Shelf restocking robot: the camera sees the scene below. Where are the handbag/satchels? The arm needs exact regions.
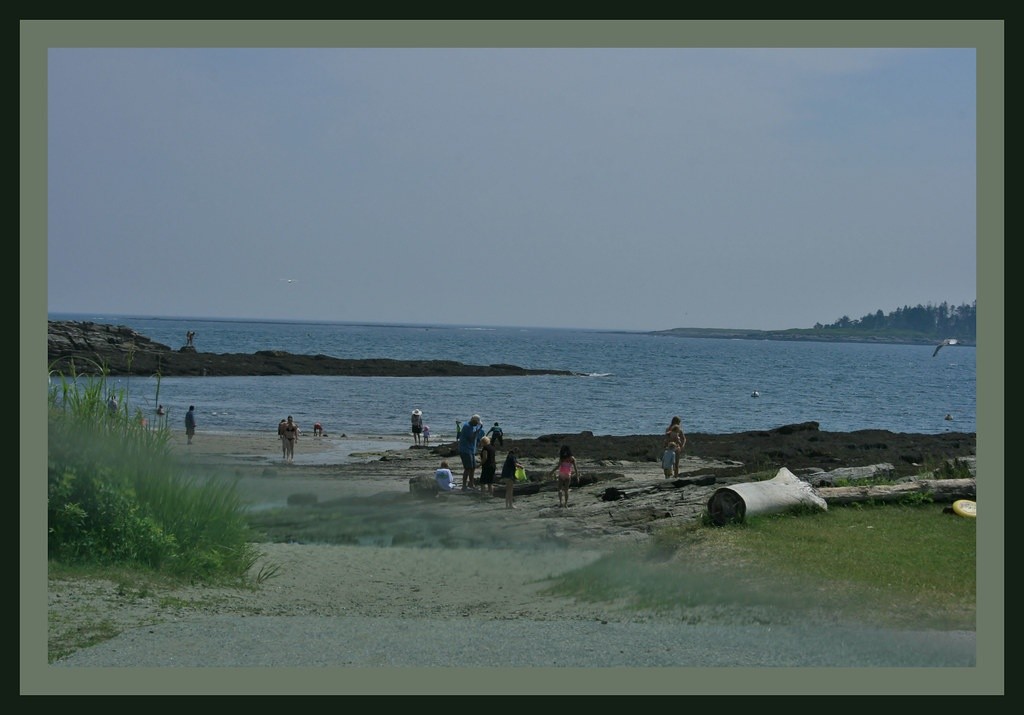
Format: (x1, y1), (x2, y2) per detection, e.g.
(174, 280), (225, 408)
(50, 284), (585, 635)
(515, 467), (527, 482)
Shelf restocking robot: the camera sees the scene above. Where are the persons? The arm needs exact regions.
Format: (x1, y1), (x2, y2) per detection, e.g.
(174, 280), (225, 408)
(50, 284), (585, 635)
(108, 396), (118, 426)
(411, 409), (504, 498)
(157, 405), (165, 415)
(313, 422), (323, 437)
(501, 447), (524, 509)
(186, 331), (195, 346)
(662, 416), (686, 479)
(549, 445), (580, 508)
(185, 405), (197, 445)
(278, 415), (302, 462)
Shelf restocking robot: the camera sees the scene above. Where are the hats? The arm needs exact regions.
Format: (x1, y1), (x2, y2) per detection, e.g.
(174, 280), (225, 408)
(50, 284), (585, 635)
(481, 436), (490, 446)
(423, 426), (430, 431)
(471, 414), (482, 425)
(666, 442), (680, 450)
(514, 447), (522, 459)
(412, 409), (422, 415)
(672, 424), (680, 433)
